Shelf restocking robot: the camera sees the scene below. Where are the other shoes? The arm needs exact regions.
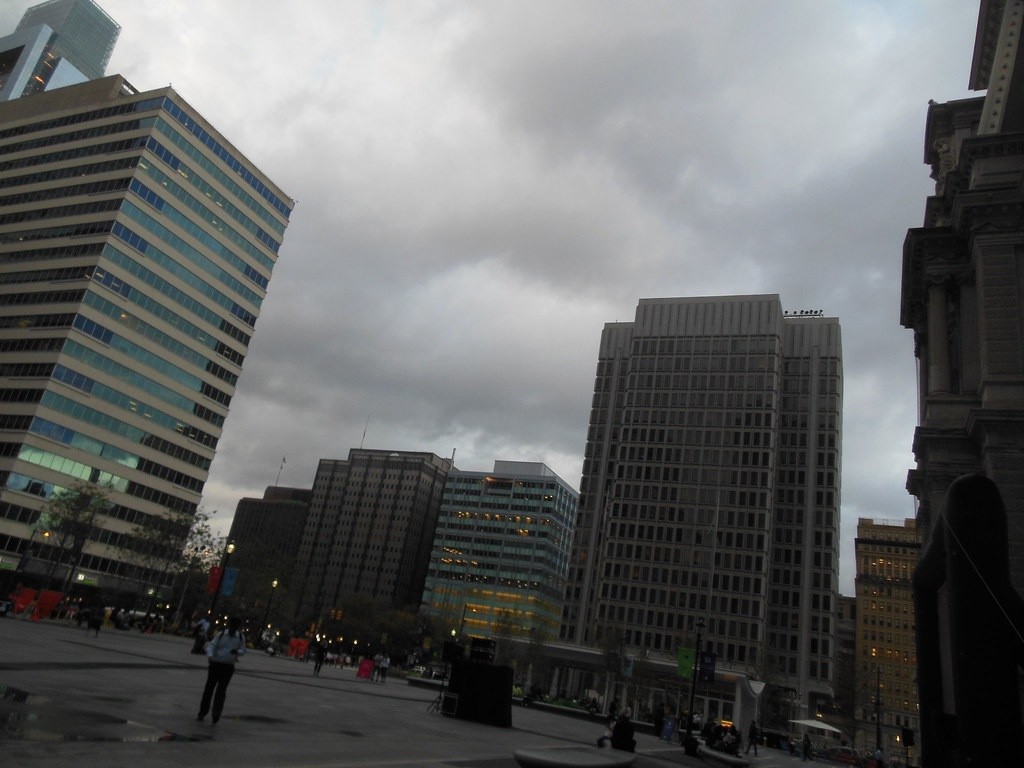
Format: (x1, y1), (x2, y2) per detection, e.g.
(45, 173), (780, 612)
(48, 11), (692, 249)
(197, 714), (204, 721)
(213, 718), (218, 724)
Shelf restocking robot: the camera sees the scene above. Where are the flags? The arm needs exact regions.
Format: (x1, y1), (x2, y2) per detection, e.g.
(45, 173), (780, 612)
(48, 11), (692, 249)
(288, 638), (312, 658)
(31, 588), (64, 621)
(208, 566), (223, 592)
(15, 588), (36, 610)
(222, 566), (240, 594)
(356, 658), (376, 680)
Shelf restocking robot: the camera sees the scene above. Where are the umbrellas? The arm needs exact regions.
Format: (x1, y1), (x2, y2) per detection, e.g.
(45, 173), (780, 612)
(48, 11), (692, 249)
(786, 717), (843, 750)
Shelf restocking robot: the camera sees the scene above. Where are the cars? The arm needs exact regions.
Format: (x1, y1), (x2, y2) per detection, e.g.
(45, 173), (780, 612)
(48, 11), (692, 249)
(758, 729), (796, 751)
(410, 665), (424, 674)
(821, 746), (860, 763)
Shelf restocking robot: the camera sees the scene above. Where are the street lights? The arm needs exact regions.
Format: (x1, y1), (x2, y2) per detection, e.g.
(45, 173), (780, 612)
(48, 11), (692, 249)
(209, 542), (235, 621)
(2, 530), (49, 601)
(687, 620), (706, 737)
(876, 670), (884, 750)
(258, 580), (278, 645)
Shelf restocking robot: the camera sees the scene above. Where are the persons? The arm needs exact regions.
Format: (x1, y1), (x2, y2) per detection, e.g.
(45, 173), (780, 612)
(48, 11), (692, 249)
(197, 615), (246, 724)
(0, 582), (390, 683)
(403, 663), (886, 768)
